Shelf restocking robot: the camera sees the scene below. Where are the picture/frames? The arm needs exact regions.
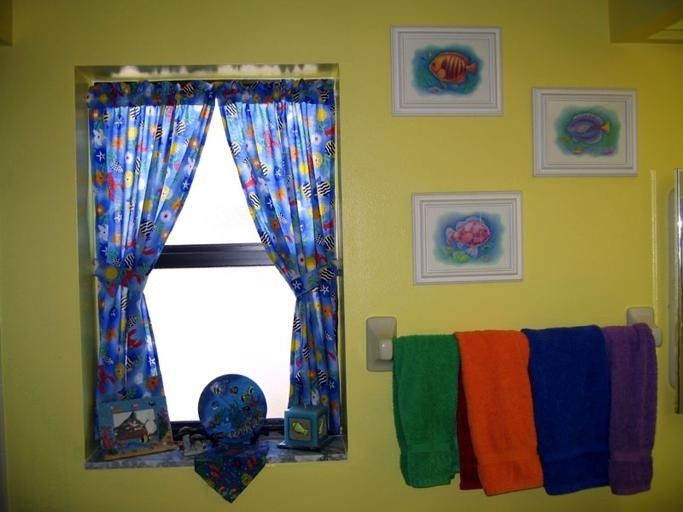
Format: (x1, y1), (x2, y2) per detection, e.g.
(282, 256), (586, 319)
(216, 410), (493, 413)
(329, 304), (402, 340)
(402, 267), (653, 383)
(532, 88), (637, 177)
(413, 191), (523, 284)
(96, 396), (176, 461)
(391, 26), (503, 116)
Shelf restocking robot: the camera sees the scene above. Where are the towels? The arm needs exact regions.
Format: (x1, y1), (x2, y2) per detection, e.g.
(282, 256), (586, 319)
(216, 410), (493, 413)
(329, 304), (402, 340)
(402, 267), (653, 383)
(600, 323), (658, 494)
(452, 330), (544, 496)
(520, 324), (611, 496)
(395, 332), (461, 488)
